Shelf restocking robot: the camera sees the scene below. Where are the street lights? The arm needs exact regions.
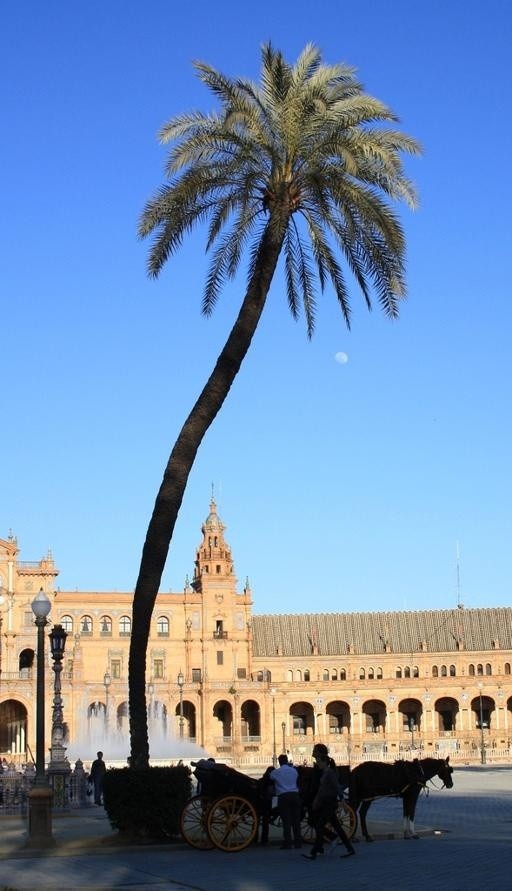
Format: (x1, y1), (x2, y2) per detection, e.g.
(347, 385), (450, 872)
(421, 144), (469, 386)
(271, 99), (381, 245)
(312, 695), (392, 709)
(178, 667), (185, 738)
(476, 679), (488, 764)
(281, 721), (287, 753)
(102, 669), (111, 733)
(23, 586), (61, 848)
(46, 623), (74, 811)
(147, 678), (155, 722)
(268, 687), (279, 769)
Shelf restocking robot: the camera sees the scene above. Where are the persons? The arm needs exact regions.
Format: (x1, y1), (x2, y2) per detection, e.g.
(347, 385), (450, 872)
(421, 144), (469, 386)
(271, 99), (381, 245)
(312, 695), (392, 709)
(268, 754), (303, 850)
(177, 760), (184, 767)
(300, 754), (357, 862)
(2, 758), (10, 773)
(1, 757), (5, 775)
(251, 765), (276, 843)
(87, 751), (109, 808)
(196, 757), (216, 827)
(306, 743), (340, 859)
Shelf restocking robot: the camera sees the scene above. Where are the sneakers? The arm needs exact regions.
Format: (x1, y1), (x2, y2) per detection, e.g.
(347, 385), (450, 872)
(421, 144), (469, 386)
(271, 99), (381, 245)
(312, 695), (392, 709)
(94, 799), (104, 807)
(340, 851), (355, 858)
(302, 854), (316, 860)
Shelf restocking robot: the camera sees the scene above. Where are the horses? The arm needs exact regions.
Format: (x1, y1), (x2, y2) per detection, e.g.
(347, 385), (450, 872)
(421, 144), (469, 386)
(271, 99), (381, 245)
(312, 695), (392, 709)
(349, 756), (453, 842)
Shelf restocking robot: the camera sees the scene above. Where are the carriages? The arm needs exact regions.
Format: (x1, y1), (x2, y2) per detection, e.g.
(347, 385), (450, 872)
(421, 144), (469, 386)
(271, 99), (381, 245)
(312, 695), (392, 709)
(178, 754), (454, 854)
